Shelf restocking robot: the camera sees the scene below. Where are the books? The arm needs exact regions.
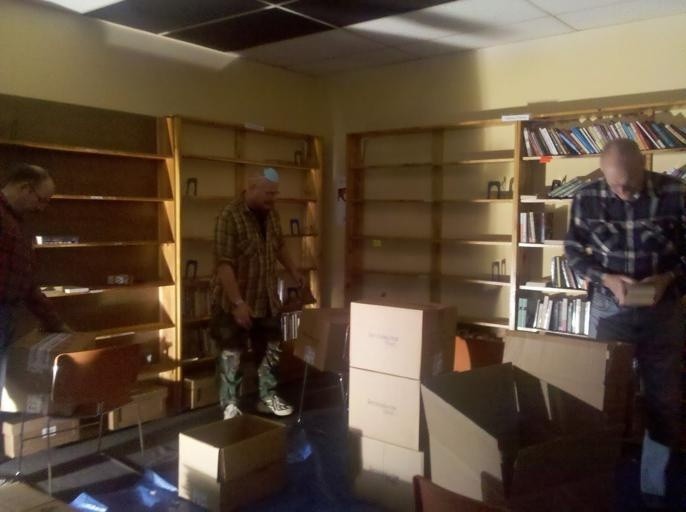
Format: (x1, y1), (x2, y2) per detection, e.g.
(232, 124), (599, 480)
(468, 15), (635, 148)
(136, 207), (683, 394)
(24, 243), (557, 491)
(522, 118), (686, 156)
(663, 167), (686, 182)
(515, 176), (601, 336)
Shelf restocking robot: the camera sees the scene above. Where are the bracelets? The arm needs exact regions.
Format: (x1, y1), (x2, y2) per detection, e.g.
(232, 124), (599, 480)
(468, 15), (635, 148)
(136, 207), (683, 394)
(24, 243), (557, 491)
(664, 270), (677, 280)
(230, 299), (244, 309)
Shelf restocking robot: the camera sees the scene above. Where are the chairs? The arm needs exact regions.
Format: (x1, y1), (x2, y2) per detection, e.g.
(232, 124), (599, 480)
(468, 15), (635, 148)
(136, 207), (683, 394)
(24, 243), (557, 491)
(12, 345), (146, 500)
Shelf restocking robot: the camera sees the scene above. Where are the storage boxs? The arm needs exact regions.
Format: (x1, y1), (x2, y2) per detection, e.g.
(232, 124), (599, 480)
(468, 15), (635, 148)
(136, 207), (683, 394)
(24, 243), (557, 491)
(1, 478), (85, 512)
(176, 412), (288, 511)
(345, 293), (633, 511)
(0, 326), (99, 418)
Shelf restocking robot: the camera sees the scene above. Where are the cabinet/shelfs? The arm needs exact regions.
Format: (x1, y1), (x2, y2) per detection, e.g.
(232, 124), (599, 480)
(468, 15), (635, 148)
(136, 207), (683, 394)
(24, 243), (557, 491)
(517, 97), (685, 341)
(175, 113), (323, 411)
(0, 92), (180, 458)
(343, 117), (518, 332)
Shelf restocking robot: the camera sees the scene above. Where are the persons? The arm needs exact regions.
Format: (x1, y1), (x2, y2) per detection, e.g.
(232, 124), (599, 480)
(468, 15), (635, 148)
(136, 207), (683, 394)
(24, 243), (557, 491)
(0, 164), (73, 398)
(561, 135), (686, 474)
(207, 171), (306, 421)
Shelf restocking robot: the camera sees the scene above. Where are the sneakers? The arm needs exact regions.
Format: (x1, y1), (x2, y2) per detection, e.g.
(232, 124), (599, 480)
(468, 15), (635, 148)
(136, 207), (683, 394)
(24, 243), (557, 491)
(255, 393), (295, 416)
(223, 403), (243, 421)
